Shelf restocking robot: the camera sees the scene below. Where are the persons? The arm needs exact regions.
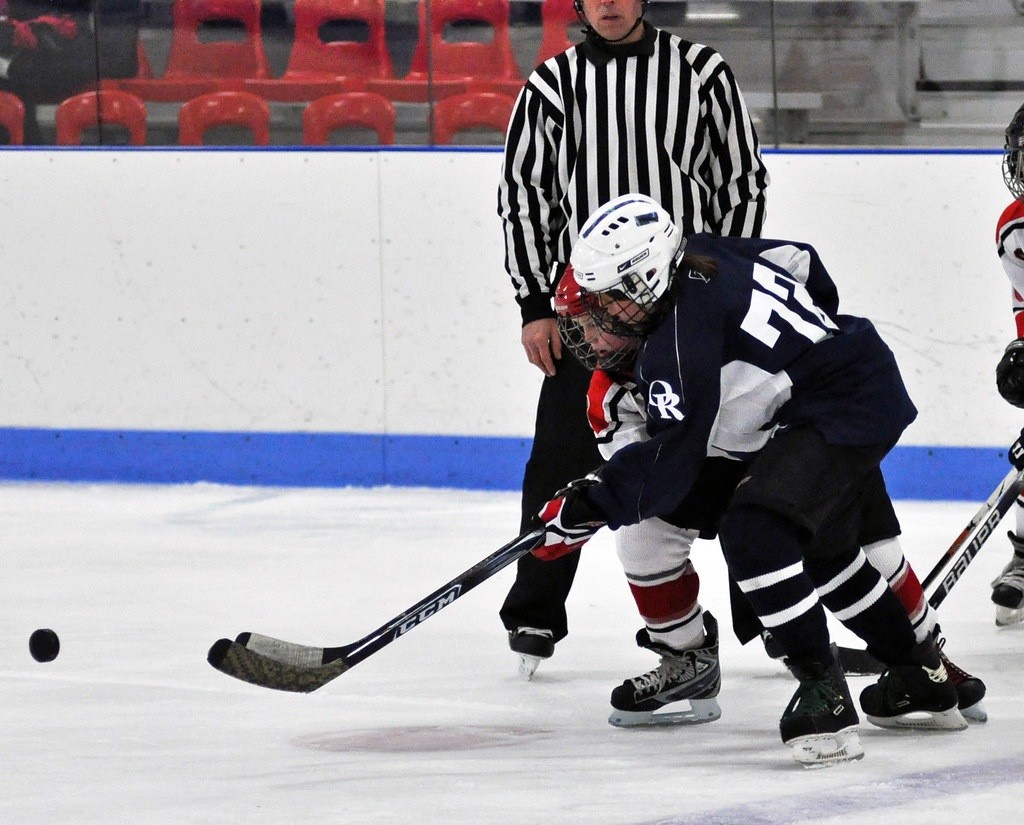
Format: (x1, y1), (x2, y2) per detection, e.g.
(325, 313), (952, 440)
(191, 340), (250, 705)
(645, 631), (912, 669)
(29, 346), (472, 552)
(498, 2), (771, 680)
(0, 0), (139, 146)
(991, 104), (1024, 627)
(533, 194), (990, 768)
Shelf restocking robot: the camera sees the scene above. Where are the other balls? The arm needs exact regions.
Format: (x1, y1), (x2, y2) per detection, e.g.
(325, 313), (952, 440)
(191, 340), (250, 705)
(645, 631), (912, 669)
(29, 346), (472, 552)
(28, 629), (59, 663)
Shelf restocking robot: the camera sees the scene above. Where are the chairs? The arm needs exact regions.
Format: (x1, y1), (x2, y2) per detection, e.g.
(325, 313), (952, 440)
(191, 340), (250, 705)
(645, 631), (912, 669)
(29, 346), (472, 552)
(0, 0), (589, 146)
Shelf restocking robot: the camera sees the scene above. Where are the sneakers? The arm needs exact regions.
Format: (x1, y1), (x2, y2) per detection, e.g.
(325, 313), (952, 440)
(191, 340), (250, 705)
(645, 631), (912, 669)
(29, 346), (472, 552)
(608, 610), (722, 728)
(858, 621), (969, 730)
(990, 531), (1024, 626)
(508, 625), (555, 682)
(779, 646), (864, 769)
(924, 623), (989, 722)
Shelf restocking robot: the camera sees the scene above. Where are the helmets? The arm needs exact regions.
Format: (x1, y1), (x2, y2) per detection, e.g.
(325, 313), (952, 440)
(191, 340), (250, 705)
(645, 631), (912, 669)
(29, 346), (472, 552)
(569, 194), (687, 336)
(556, 267), (641, 371)
(1001, 102), (1024, 202)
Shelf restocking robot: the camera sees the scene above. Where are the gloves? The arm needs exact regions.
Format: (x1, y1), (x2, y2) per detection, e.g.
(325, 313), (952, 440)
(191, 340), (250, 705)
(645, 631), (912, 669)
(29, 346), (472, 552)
(995, 336), (1024, 412)
(530, 467), (607, 562)
(1008, 426), (1024, 473)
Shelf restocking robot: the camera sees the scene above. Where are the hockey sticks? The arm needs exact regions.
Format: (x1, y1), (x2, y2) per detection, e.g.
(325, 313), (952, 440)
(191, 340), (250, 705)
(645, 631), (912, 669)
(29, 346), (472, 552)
(208, 523), (549, 694)
(782, 465), (1021, 679)
(236, 518), (546, 667)
(832, 472), (1024, 674)
